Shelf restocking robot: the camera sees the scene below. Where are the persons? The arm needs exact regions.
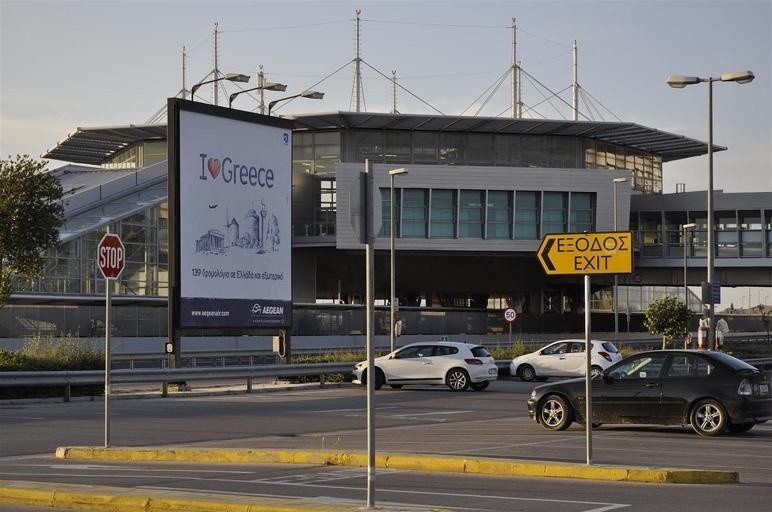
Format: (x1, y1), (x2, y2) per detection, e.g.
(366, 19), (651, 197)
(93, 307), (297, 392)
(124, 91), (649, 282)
(682, 314), (729, 350)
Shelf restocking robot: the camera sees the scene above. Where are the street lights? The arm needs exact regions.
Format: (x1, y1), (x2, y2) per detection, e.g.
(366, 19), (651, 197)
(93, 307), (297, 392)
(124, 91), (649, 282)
(613, 175), (629, 347)
(661, 68), (756, 357)
(385, 167), (413, 357)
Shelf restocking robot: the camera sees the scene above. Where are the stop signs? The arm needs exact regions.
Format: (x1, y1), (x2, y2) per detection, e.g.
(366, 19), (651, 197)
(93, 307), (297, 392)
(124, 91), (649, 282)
(95, 232), (127, 282)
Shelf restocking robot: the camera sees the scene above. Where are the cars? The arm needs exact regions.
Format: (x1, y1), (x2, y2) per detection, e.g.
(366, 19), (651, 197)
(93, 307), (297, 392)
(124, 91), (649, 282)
(349, 339), (501, 394)
(525, 347), (772, 439)
(508, 337), (625, 385)
